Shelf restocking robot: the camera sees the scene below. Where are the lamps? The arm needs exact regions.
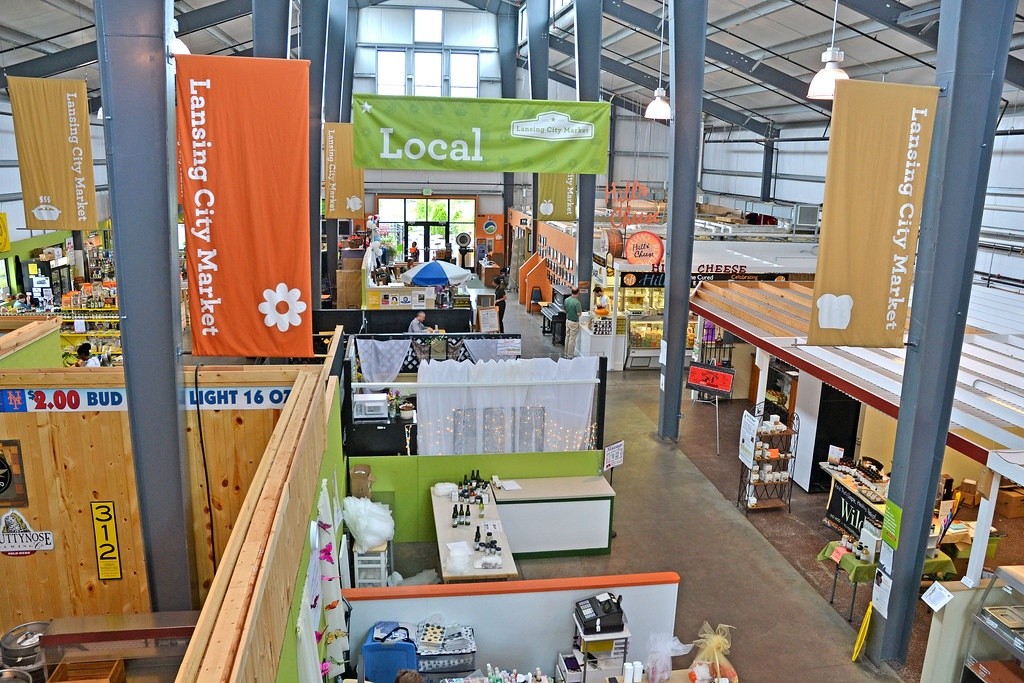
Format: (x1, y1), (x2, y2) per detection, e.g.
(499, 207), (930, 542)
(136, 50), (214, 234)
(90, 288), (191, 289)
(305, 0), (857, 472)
(172, 20), (193, 74)
(643, 0), (671, 119)
(97, 107), (103, 119)
(804, 0), (850, 99)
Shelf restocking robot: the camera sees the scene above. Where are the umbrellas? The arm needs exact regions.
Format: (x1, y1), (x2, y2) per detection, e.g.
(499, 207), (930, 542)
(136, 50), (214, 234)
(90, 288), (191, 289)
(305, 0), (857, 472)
(401, 259), (471, 286)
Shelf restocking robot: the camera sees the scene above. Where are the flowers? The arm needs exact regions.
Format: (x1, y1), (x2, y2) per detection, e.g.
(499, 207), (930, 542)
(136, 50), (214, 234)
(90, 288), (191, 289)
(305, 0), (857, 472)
(389, 389), (414, 410)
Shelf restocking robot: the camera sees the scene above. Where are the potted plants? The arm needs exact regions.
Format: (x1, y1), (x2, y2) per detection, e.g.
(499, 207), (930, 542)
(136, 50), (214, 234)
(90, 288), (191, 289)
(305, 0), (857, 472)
(386, 248), (399, 266)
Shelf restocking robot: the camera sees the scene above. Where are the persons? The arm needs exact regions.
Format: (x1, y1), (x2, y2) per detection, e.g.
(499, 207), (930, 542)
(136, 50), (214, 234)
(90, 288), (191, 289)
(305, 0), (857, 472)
(395, 670), (423, 683)
(410, 242), (420, 262)
(77, 343), (101, 367)
(593, 287), (610, 310)
(492, 277), (507, 333)
(14, 293), (31, 310)
(408, 311), (433, 333)
(564, 288), (582, 359)
(436, 285), (443, 293)
(403, 259), (414, 272)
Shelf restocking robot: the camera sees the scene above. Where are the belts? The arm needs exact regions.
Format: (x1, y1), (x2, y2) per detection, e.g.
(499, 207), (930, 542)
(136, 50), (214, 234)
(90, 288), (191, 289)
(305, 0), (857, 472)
(567, 318), (578, 322)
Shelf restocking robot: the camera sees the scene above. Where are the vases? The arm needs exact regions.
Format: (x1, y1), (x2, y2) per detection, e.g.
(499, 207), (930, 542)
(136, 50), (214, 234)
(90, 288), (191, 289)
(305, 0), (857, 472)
(389, 399), (413, 420)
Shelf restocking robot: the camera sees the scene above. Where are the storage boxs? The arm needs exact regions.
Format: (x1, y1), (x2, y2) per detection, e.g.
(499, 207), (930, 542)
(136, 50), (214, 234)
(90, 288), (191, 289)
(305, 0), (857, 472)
(938, 474), (1023, 559)
(46, 656), (127, 683)
(350, 464), (375, 497)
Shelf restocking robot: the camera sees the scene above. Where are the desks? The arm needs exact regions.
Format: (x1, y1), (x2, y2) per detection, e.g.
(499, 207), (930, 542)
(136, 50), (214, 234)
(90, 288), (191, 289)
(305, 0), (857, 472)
(39, 610), (202, 683)
(429, 475), (614, 584)
(479, 260), (500, 287)
(818, 462), (960, 622)
(343, 396), (418, 456)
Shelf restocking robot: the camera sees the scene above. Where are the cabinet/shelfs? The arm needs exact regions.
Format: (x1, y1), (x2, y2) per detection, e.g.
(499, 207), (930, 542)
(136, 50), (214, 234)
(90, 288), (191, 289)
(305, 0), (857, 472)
(351, 538), (389, 589)
(62, 248), (121, 366)
(579, 284), (697, 369)
(554, 612), (632, 683)
(959, 564), (1024, 682)
(737, 401), (800, 516)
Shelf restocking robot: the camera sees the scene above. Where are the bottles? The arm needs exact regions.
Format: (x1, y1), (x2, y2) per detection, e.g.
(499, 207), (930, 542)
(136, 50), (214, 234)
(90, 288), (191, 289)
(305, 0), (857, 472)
(837, 456), (883, 492)
(458, 469), (490, 504)
(119, 336), (122, 348)
(452, 504), (470, 527)
(842, 516), (883, 563)
(475, 526), (501, 555)
(87, 296), (102, 309)
(479, 498), (484, 518)
